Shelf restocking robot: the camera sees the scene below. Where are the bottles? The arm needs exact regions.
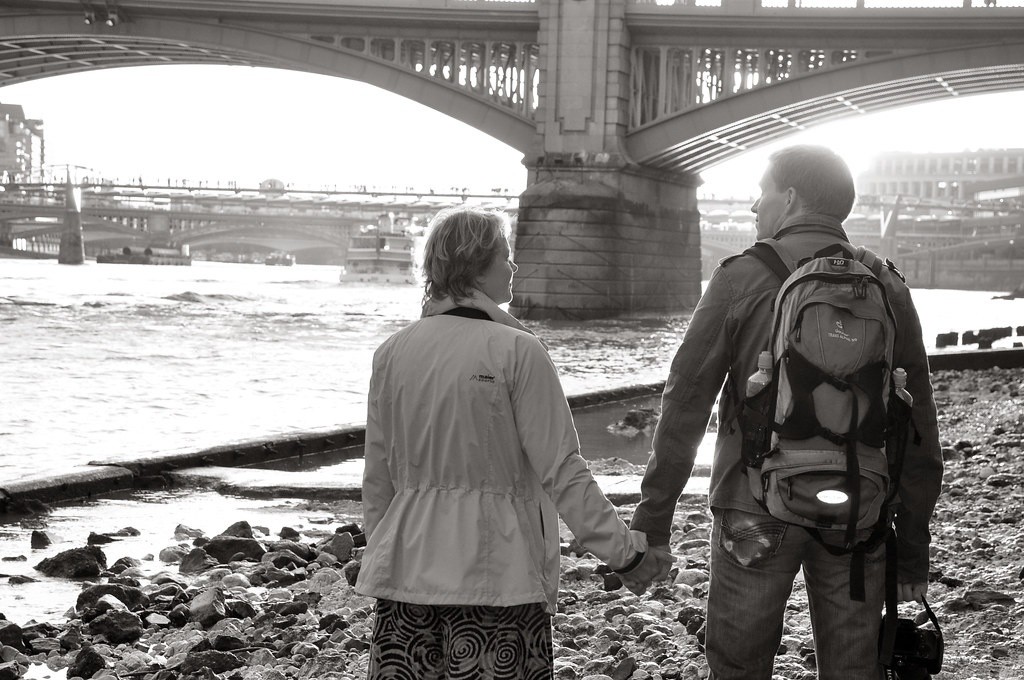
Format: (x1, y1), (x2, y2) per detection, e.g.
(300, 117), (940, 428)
(742, 351), (774, 468)
(887, 367), (913, 466)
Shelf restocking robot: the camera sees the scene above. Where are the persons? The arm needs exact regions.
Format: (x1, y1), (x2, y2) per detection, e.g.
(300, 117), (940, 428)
(352, 209), (679, 680)
(620, 144), (944, 680)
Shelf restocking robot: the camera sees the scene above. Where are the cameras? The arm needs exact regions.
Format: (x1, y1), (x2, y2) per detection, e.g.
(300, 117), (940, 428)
(878, 593), (944, 680)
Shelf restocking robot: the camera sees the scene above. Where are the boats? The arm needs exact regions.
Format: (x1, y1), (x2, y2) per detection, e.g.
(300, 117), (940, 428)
(264, 254), (296, 267)
(338, 214), (428, 288)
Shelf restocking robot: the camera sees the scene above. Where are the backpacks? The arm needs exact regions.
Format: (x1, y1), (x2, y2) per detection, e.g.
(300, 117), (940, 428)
(743, 241), (905, 528)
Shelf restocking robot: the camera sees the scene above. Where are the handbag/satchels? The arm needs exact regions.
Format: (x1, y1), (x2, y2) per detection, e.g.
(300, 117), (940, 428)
(891, 595), (945, 675)
